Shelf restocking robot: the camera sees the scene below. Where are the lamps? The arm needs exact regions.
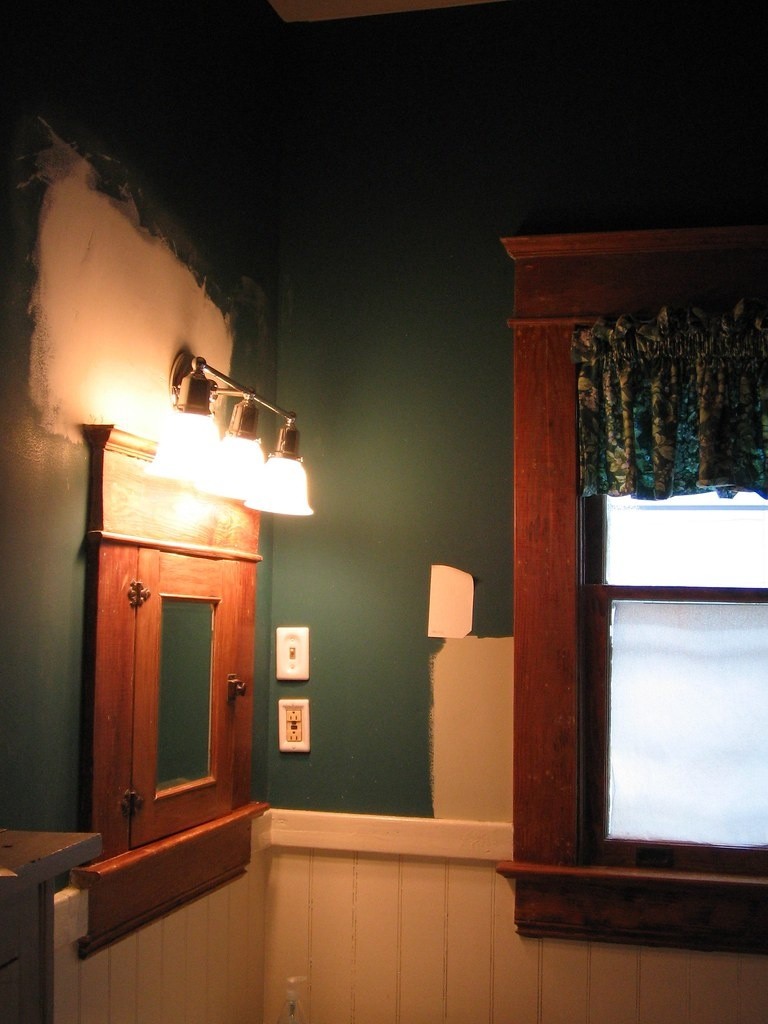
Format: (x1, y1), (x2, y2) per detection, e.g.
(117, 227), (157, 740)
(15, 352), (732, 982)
(144, 358), (319, 519)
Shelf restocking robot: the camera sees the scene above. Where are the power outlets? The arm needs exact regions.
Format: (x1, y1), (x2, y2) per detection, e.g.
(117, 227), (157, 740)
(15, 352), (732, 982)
(276, 698), (312, 754)
